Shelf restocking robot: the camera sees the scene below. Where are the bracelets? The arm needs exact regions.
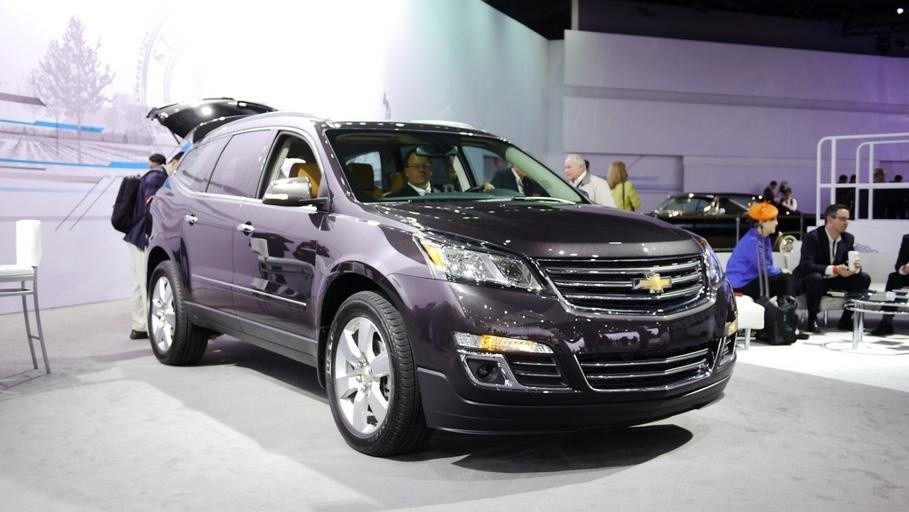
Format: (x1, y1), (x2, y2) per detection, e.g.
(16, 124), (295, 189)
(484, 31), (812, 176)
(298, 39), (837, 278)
(833, 265), (838, 274)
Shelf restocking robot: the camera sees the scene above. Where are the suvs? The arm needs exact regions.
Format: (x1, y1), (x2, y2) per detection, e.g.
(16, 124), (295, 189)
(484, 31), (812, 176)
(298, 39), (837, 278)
(141, 97), (738, 457)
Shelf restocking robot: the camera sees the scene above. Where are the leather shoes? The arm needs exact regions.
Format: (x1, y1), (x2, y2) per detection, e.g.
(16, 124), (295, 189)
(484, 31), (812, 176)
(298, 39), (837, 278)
(130, 330), (149, 340)
(796, 331), (809, 339)
(807, 320), (820, 334)
(872, 322), (894, 335)
(838, 319), (853, 331)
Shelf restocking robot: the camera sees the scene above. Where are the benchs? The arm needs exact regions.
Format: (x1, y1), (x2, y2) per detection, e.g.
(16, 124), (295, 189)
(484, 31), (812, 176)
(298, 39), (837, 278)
(716, 250), (909, 349)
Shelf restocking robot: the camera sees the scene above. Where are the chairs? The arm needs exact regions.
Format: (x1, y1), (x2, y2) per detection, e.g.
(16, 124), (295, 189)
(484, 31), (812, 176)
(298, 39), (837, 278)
(347, 163), (383, 199)
(382, 172), (407, 199)
(289, 164), (321, 198)
(0, 220), (52, 374)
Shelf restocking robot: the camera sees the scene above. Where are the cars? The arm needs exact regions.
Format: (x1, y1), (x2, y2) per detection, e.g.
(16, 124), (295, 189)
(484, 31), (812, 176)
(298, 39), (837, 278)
(642, 192), (825, 253)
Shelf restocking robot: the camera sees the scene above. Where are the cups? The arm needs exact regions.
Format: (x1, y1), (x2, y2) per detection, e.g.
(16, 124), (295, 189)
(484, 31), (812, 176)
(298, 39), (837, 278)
(847, 250), (861, 274)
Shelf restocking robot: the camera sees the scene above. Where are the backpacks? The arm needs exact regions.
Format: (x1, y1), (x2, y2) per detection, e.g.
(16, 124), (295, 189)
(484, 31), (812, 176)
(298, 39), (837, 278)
(111, 170), (163, 233)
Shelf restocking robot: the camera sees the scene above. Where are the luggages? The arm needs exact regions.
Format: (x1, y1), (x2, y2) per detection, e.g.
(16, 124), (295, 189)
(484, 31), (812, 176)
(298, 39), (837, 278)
(755, 222), (798, 345)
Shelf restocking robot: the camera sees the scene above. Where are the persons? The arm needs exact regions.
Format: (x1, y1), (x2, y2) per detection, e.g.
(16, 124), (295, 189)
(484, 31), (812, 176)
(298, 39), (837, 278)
(491, 163), (535, 197)
(563, 154), (616, 208)
(836, 168), (909, 219)
(385, 148), (495, 197)
(763, 180), (797, 212)
(870, 233), (909, 336)
(149, 152), (166, 169)
(123, 151), (186, 341)
(792, 204), (871, 333)
(607, 160), (639, 212)
(723, 202), (809, 341)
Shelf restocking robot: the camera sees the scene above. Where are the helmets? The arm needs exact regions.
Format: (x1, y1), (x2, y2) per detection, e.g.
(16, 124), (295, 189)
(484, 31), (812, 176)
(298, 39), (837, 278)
(748, 202), (779, 222)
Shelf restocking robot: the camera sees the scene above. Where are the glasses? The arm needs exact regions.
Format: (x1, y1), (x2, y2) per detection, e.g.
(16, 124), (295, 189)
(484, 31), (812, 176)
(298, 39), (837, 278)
(408, 162), (431, 170)
